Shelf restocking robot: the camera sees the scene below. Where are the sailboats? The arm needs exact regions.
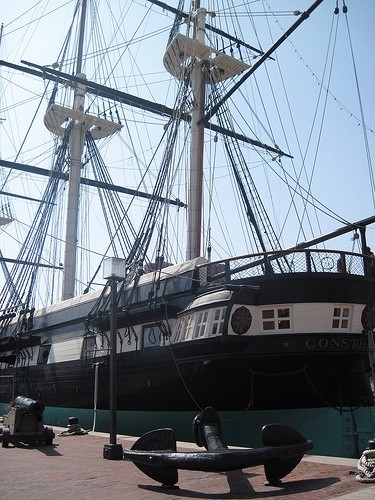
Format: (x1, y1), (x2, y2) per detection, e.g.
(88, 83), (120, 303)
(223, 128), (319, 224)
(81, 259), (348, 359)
(0, 0), (375, 403)
